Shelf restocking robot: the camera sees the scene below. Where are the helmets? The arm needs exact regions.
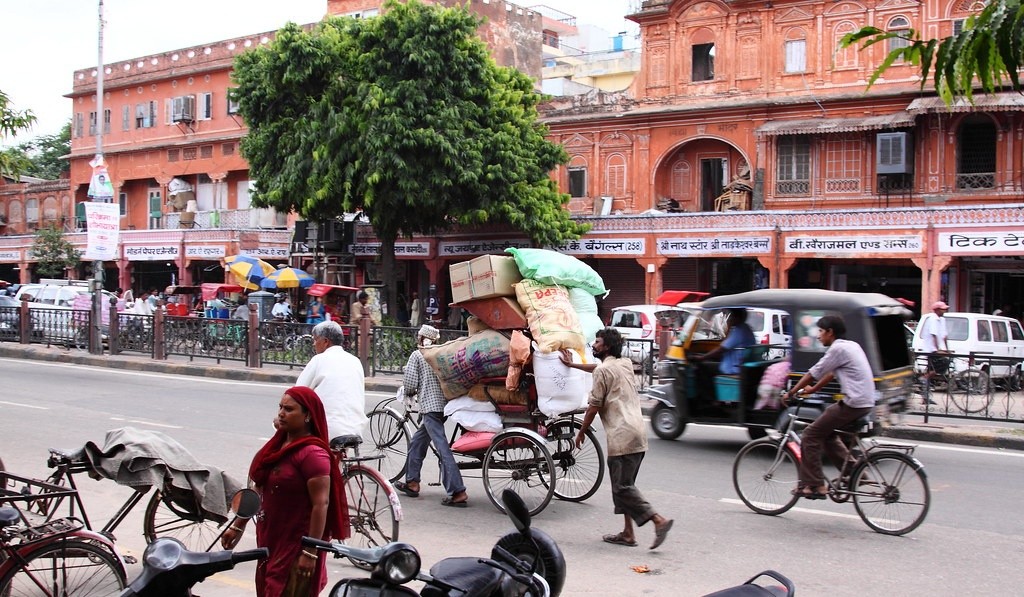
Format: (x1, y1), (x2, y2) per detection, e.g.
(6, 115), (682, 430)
(275, 292), (287, 302)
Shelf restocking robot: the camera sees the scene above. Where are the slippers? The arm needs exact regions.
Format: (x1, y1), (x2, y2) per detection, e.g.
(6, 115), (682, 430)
(603, 533), (638, 546)
(441, 496), (467, 506)
(395, 480), (420, 497)
(650, 520), (673, 550)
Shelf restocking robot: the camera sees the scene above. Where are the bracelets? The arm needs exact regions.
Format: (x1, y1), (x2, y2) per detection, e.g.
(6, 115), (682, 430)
(229, 525), (244, 533)
(788, 390), (793, 396)
(301, 549), (319, 559)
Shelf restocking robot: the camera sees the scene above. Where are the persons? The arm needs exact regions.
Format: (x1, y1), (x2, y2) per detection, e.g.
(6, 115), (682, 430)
(912, 301), (951, 405)
(989, 305), (1010, 342)
(559, 329), (674, 550)
(109, 286), (460, 352)
(781, 315), (875, 501)
(688, 306), (758, 417)
(221, 386), (351, 597)
(273, 321), (370, 545)
(393, 324), (468, 507)
(96, 175), (111, 195)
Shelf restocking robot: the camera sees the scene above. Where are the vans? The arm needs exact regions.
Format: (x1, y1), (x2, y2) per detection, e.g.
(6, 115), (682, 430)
(12, 279), (145, 351)
(910, 312), (1024, 391)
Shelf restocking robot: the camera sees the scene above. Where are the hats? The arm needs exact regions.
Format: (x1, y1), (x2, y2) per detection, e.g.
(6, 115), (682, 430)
(933, 301), (949, 309)
(992, 309), (1003, 315)
(418, 324), (440, 340)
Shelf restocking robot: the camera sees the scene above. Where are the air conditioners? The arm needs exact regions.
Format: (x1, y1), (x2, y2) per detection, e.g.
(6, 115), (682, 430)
(172, 96), (193, 122)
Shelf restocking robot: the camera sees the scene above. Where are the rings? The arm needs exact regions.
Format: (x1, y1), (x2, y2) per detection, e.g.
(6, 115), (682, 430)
(306, 571), (311, 577)
(297, 570), (302, 575)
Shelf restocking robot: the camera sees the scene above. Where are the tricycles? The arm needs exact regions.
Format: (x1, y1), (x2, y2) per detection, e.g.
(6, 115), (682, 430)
(354, 378), (605, 519)
(647, 287), (913, 445)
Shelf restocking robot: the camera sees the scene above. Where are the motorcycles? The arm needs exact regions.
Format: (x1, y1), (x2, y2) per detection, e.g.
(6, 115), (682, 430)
(477, 489), (795, 597)
(300, 528), (567, 597)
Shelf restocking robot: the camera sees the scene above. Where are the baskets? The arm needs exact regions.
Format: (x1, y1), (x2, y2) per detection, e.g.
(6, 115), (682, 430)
(932, 357), (950, 372)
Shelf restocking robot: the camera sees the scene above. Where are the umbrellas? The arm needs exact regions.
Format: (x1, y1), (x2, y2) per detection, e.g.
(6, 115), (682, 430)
(220, 254), (316, 313)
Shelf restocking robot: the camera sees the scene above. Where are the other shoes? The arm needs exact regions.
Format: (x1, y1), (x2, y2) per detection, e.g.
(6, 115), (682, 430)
(923, 399), (937, 404)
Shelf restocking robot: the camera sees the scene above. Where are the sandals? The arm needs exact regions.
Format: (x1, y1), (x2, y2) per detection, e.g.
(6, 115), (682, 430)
(792, 485), (826, 499)
(842, 474), (869, 486)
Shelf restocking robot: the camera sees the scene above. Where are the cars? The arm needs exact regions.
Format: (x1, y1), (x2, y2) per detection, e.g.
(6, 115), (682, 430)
(903, 323), (915, 351)
(742, 307), (793, 360)
(0, 295), (46, 343)
(607, 304), (695, 376)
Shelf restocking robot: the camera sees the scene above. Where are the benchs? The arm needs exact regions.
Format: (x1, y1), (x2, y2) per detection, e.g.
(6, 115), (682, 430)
(712, 345), (788, 403)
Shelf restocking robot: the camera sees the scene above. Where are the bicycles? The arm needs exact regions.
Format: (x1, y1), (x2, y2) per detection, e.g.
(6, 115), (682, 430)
(72, 310), (317, 357)
(0, 514), (129, 597)
(908, 347), (995, 414)
(22, 443), (243, 554)
(327, 433), (404, 571)
(732, 378), (931, 535)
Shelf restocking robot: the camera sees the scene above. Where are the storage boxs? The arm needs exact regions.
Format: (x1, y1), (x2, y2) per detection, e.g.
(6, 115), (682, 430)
(449, 254), (521, 303)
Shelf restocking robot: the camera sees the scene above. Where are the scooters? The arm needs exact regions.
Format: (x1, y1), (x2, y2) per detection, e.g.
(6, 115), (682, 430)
(115, 489), (270, 597)
